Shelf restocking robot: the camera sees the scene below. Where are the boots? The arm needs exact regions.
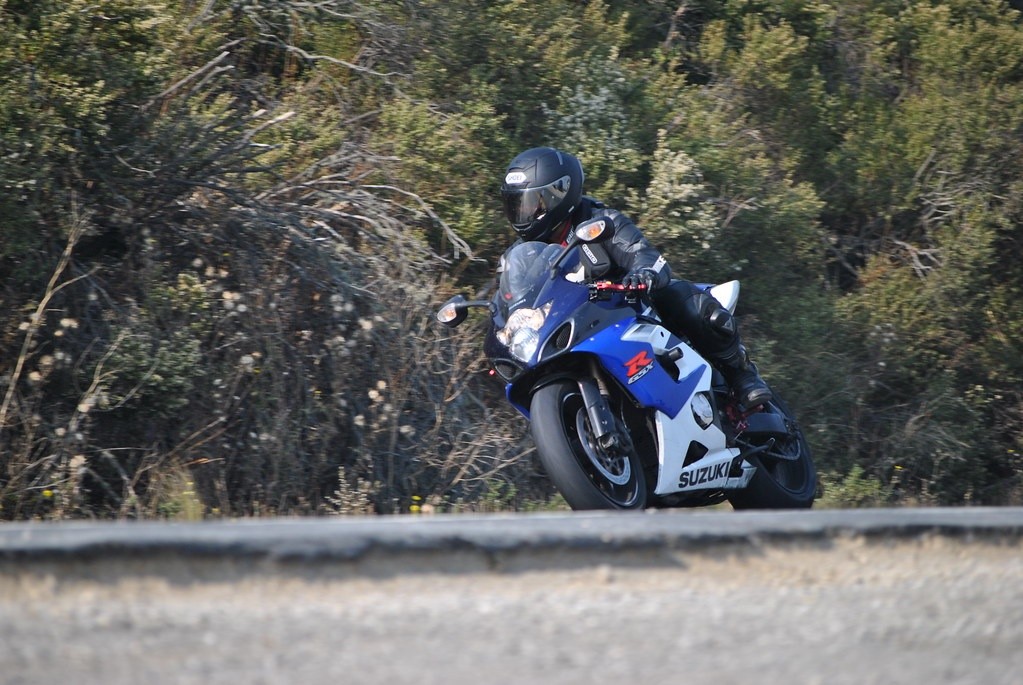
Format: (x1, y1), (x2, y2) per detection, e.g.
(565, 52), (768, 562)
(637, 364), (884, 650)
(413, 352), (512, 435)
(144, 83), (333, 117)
(704, 330), (773, 409)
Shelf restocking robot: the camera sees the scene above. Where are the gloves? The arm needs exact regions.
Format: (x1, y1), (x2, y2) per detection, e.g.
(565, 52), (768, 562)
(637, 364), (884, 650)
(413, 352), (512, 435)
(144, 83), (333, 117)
(622, 267), (660, 296)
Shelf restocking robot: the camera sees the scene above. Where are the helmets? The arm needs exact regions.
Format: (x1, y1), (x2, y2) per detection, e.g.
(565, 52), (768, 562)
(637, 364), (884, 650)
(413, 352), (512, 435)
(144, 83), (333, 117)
(500, 146), (586, 242)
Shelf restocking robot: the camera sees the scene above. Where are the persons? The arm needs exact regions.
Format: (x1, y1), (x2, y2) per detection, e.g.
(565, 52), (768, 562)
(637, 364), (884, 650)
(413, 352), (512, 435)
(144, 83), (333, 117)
(479, 147), (772, 405)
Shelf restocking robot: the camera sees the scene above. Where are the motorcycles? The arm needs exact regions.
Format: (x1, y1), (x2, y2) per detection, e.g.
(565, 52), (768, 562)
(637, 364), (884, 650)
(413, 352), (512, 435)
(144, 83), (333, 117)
(436, 216), (816, 509)
